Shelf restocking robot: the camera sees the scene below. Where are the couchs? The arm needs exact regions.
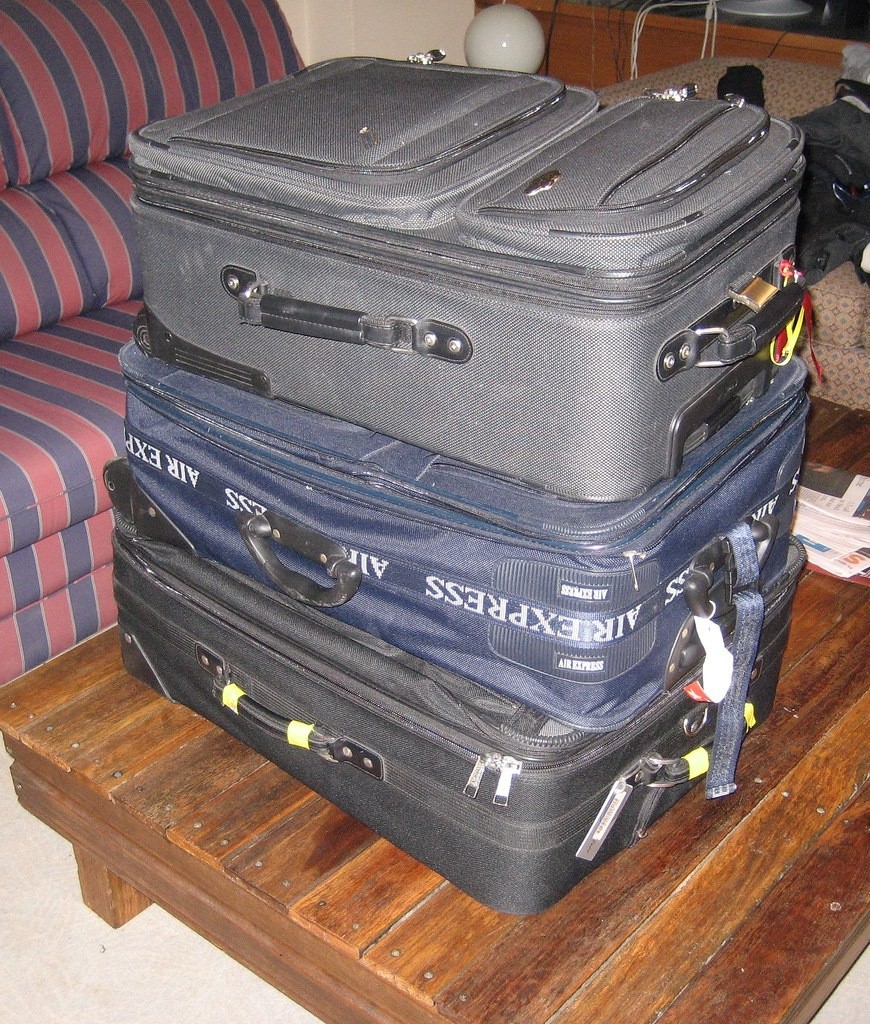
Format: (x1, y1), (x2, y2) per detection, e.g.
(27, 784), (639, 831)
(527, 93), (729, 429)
(0, 0), (339, 688)
(594, 54), (870, 417)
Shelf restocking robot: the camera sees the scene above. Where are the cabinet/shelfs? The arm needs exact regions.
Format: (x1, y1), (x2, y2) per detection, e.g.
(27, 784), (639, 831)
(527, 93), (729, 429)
(472, 0), (870, 96)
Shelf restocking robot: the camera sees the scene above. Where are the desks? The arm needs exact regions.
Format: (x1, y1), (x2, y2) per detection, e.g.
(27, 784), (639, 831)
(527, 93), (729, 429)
(0, 392), (870, 1024)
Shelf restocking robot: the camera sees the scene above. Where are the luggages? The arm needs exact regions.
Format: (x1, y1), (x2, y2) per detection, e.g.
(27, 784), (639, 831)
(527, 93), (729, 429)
(110, 531), (796, 918)
(127, 57), (807, 492)
(120, 347), (806, 731)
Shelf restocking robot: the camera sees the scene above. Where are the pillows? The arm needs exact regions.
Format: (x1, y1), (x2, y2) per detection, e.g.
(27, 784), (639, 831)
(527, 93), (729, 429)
(0, 0), (306, 347)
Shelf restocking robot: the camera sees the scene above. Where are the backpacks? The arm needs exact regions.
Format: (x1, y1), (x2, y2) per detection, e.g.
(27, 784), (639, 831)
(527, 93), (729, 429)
(790, 78), (870, 280)
